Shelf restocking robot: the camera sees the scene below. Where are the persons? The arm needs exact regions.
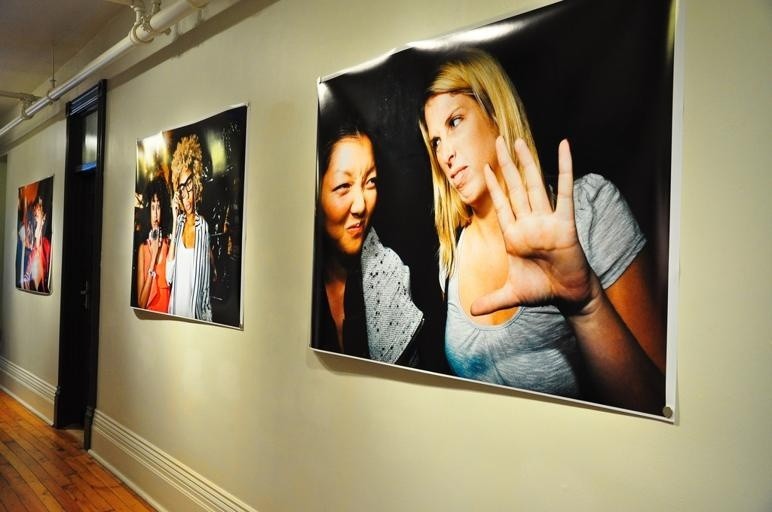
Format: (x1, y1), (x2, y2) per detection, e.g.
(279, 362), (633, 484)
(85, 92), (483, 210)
(135, 174), (174, 315)
(415, 45), (666, 417)
(17, 195), (52, 295)
(313, 109), (426, 370)
(164, 134), (215, 324)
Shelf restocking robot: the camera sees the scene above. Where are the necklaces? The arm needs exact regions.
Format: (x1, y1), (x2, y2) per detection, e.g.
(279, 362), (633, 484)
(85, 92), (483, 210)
(186, 224), (195, 240)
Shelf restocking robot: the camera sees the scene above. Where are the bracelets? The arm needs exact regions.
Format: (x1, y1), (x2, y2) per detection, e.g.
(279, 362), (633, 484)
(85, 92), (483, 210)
(147, 270), (157, 277)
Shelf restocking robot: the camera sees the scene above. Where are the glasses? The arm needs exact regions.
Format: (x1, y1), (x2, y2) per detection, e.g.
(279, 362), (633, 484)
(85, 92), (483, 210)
(177, 174), (196, 200)
